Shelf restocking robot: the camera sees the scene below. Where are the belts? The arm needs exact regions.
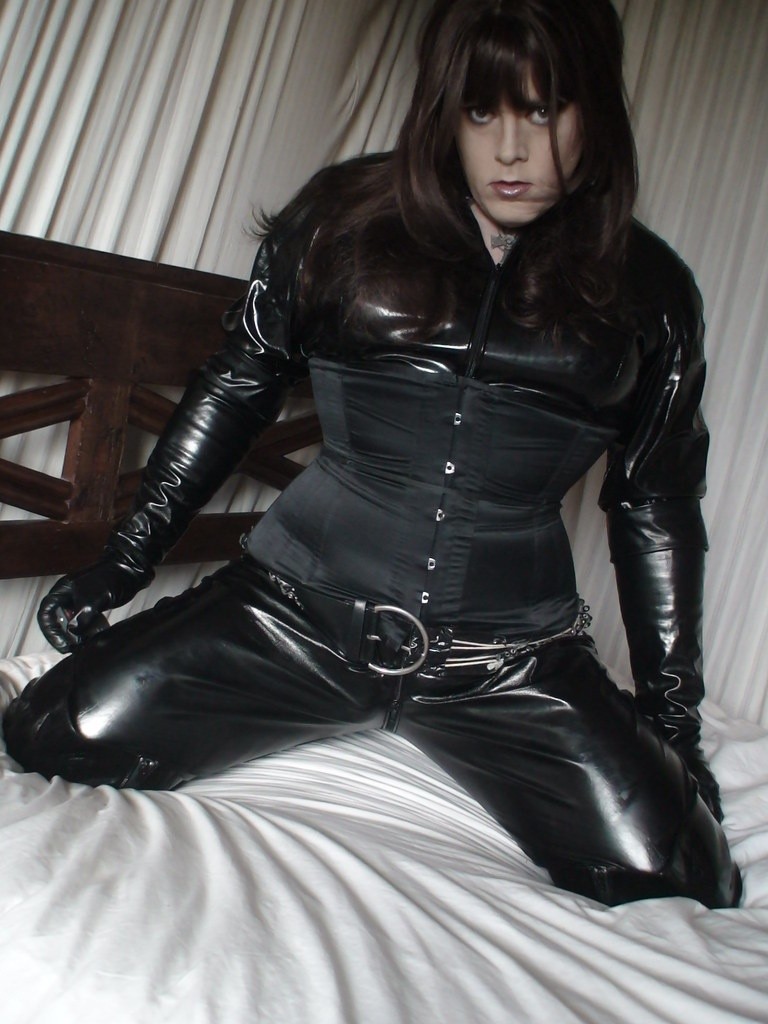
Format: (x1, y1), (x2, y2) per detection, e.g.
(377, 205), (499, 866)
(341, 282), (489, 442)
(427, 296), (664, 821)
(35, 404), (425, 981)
(262, 569), (430, 676)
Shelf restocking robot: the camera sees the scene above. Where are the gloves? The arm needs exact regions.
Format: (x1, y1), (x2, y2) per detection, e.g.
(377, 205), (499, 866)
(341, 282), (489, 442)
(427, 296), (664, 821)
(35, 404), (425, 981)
(607, 497), (726, 826)
(38, 336), (293, 650)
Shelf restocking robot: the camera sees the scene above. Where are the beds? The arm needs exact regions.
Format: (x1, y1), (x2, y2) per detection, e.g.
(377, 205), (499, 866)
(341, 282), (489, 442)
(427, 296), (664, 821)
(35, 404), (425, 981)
(1, 230), (767, 1024)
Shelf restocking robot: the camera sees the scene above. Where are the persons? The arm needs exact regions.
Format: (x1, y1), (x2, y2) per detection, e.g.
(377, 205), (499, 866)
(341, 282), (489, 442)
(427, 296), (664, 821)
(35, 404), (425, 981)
(0, 0), (748, 919)
